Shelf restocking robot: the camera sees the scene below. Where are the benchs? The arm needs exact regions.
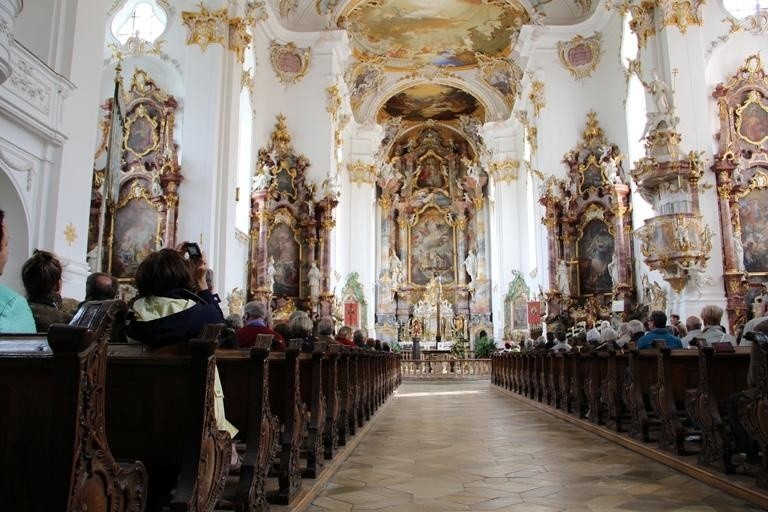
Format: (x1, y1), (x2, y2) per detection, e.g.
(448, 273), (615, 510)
(0, 299), (402, 512)
(489, 332), (768, 488)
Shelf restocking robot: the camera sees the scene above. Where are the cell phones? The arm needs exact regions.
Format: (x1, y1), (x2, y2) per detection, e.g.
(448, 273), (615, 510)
(185, 242), (202, 262)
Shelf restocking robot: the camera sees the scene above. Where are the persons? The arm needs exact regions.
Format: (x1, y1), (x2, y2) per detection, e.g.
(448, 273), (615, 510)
(671, 257), (709, 289)
(561, 194), (573, 218)
(585, 235), (609, 285)
(465, 159), (482, 185)
(399, 178), (408, 191)
(408, 212), (417, 228)
(264, 255), (277, 295)
(463, 246), (479, 285)
(606, 251), (623, 293)
(266, 148), (279, 167)
(379, 160), (400, 189)
(445, 211), (454, 223)
(0, 207), (224, 348)
(461, 190), (472, 204)
(494, 306), (768, 355)
(447, 136), (457, 153)
(306, 260), (322, 302)
(635, 62), (675, 145)
(303, 200), (317, 220)
(603, 152), (622, 186)
(387, 248), (404, 286)
(220, 297), (394, 353)
(391, 193), (400, 208)
(554, 256), (570, 298)
(741, 108), (764, 141)
